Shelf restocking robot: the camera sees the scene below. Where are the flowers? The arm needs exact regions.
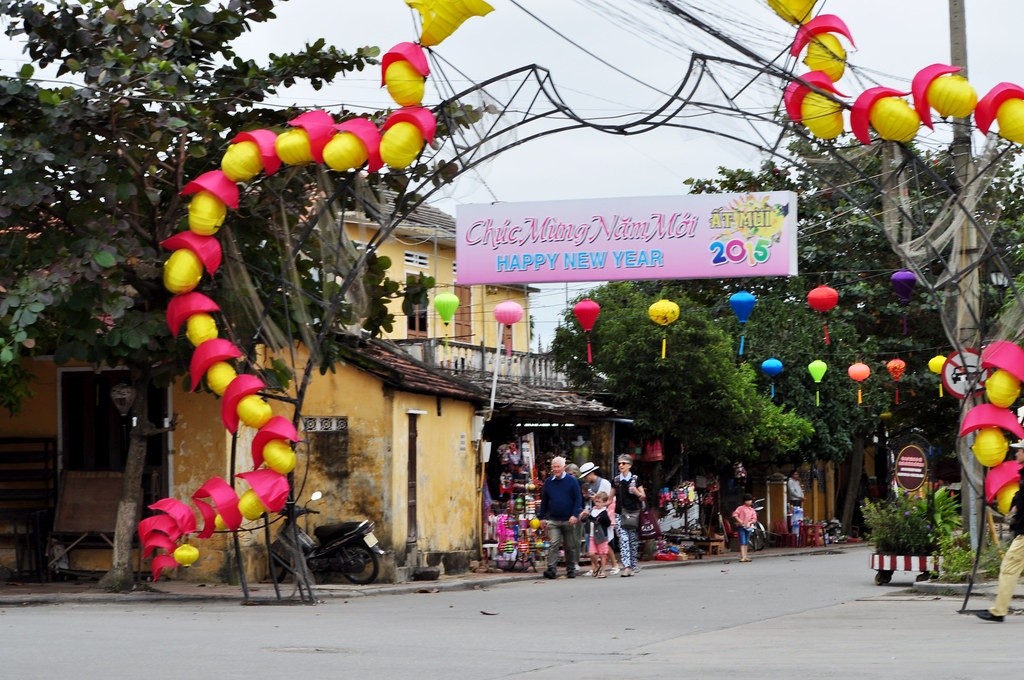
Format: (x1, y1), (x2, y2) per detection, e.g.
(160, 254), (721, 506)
(860, 488), (962, 556)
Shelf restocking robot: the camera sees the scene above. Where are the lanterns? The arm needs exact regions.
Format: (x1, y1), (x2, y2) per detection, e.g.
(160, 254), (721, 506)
(967, 369), (1020, 514)
(157, 59), (436, 567)
(890, 271), (917, 306)
(808, 285), (838, 312)
(759, 354), (948, 380)
(730, 290), (756, 324)
(646, 298), (682, 330)
(572, 297), (600, 333)
(494, 299), (525, 330)
(432, 291), (461, 328)
(803, 33), (1024, 149)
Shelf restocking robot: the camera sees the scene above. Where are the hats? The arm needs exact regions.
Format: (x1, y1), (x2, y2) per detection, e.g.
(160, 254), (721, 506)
(579, 461), (599, 479)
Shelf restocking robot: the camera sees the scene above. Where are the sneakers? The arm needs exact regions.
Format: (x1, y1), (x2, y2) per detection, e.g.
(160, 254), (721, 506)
(544, 570), (556, 579)
(567, 571), (576, 578)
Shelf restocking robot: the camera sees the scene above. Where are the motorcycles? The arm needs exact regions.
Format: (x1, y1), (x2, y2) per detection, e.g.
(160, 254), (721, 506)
(269, 491), (384, 585)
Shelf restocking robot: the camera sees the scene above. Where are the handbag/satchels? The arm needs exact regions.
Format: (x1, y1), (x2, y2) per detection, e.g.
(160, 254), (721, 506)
(621, 507), (640, 530)
(638, 509), (662, 540)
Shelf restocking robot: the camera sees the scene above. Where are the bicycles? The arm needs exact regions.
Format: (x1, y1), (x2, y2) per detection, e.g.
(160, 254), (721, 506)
(748, 498), (767, 552)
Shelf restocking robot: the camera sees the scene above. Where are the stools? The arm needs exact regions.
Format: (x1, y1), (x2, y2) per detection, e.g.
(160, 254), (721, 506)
(696, 542), (721, 555)
(804, 524), (826, 548)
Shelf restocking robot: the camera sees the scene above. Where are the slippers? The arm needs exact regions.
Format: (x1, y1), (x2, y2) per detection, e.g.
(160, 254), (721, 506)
(598, 575), (606, 578)
(592, 565), (601, 577)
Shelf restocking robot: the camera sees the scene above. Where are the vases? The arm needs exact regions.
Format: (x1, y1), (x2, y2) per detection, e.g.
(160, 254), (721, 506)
(869, 553), (943, 585)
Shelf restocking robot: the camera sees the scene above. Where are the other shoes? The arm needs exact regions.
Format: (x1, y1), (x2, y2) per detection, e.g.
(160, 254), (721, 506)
(631, 566), (639, 572)
(738, 558), (751, 563)
(620, 569), (634, 577)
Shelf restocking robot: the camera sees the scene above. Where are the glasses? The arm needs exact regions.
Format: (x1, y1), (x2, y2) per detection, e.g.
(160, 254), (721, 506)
(618, 462), (630, 466)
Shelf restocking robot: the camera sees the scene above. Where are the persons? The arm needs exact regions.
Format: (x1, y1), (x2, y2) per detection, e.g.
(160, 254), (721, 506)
(731, 494), (758, 563)
(602, 454), (645, 577)
(579, 461), (621, 576)
(976, 439), (1024, 623)
(787, 468), (808, 535)
(538, 456), (582, 579)
(583, 491), (611, 579)
